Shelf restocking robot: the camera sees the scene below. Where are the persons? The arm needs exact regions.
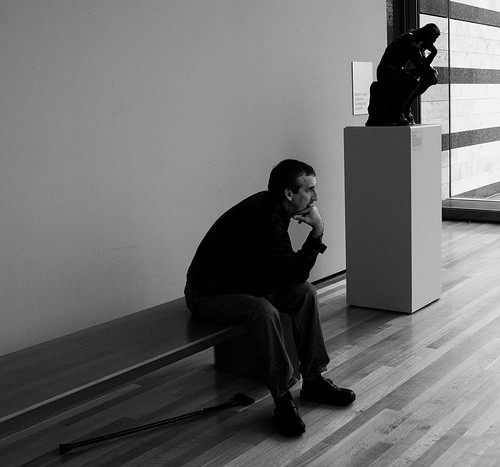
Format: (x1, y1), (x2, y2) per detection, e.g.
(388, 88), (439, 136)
(185, 159), (357, 437)
(377, 23), (441, 126)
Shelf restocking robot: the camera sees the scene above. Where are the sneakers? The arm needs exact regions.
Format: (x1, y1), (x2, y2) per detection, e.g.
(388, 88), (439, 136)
(300, 378), (356, 406)
(274, 390), (306, 434)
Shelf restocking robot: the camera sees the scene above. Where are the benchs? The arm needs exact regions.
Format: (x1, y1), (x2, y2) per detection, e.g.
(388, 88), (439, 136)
(0, 296), (256, 440)
